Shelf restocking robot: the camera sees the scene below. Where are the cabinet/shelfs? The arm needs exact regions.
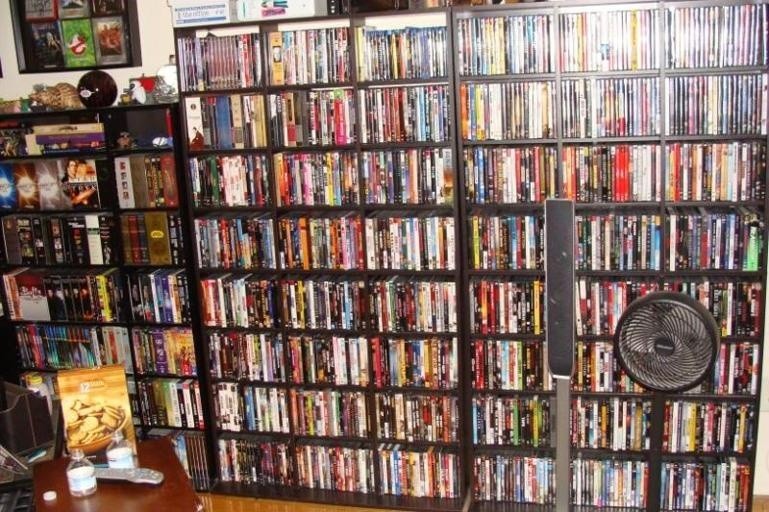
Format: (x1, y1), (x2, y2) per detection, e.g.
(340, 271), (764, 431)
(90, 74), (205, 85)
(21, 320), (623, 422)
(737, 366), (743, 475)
(0, 102), (211, 492)
(173, 7), (466, 512)
(451, 1), (769, 512)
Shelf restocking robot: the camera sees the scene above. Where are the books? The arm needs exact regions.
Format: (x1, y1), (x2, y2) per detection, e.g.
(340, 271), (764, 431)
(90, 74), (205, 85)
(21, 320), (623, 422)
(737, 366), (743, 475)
(25, 21), (64, 70)
(664, 276), (763, 338)
(363, 146), (453, 208)
(365, 210), (456, 271)
(276, 211), (364, 273)
(561, 77), (661, 139)
(289, 388), (368, 438)
(273, 150), (360, 206)
(356, 85), (451, 144)
(14, 324), (134, 375)
(91, 15), (130, 66)
(123, 268), (192, 325)
(206, 330), (286, 384)
(1, 212), (120, 267)
(570, 458), (648, 509)
(463, 144), (559, 205)
(374, 390), (464, 444)
(93, 0), (127, 16)
(664, 2), (769, 70)
(562, 142), (662, 202)
(120, 211), (189, 267)
(571, 341), (648, 394)
(470, 339), (556, 391)
(281, 274), (365, 331)
(2, 267), (123, 324)
(468, 276), (544, 336)
(195, 212), (277, 271)
(472, 393), (557, 449)
(295, 445), (375, 495)
(263, 27), (351, 87)
(218, 440), (294, 488)
(146, 429), (211, 492)
(664, 73), (769, 135)
(354, 25), (446, 83)
(132, 325), (198, 376)
(24, 0), (57, 22)
(558, 9), (660, 73)
(60, 18), (96, 67)
(378, 444), (468, 499)
(57, 365), (138, 461)
(569, 395), (652, 452)
(662, 400), (755, 453)
(574, 211), (661, 274)
(287, 332), (369, 388)
(177, 33), (263, 92)
(58, 0), (93, 18)
(212, 382), (290, 435)
(266, 89), (356, 148)
(114, 153), (178, 209)
(466, 211), (544, 270)
(200, 273), (280, 329)
(137, 377), (206, 431)
(184, 92), (268, 152)
(23, 123), (106, 156)
(368, 276), (457, 334)
(190, 154), (271, 211)
(460, 80), (557, 143)
(676, 341), (759, 395)
(574, 276), (659, 337)
(371, 336), (459, 391)
(456, 14), (556, 77)
(662, 208), (766, 272)
(471, 454), (556, 507)
(664, 141), (769, 203)
(1, 157), (108, 213)
(659, 457), (750, 512)
(126, 377), (140, 431)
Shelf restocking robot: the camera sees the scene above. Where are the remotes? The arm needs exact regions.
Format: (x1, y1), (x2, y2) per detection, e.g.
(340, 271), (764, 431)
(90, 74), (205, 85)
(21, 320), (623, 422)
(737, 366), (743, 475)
(94, 467), (164, 485)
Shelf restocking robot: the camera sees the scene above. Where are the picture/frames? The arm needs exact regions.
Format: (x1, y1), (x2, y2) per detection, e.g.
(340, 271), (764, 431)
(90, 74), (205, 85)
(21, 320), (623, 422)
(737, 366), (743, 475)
(8, 0), (142, 74)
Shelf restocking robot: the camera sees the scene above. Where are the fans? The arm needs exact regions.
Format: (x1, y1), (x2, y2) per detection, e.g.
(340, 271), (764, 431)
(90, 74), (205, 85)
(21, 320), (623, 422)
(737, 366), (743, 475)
(613, 291), (721, 512)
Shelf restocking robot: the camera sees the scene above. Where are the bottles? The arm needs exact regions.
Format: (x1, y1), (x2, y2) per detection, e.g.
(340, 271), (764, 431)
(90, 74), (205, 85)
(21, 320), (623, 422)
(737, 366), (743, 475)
(104, 431), (139, 478)
(68, 447), (100, 498)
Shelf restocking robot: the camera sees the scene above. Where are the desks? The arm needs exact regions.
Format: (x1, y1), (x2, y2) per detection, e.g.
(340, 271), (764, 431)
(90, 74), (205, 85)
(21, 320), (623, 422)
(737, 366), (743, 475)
(30, 438), (208, 512)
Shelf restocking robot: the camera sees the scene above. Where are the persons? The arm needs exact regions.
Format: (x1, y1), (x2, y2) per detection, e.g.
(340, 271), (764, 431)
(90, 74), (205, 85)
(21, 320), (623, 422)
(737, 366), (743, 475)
(60, 161), (97, 208)
